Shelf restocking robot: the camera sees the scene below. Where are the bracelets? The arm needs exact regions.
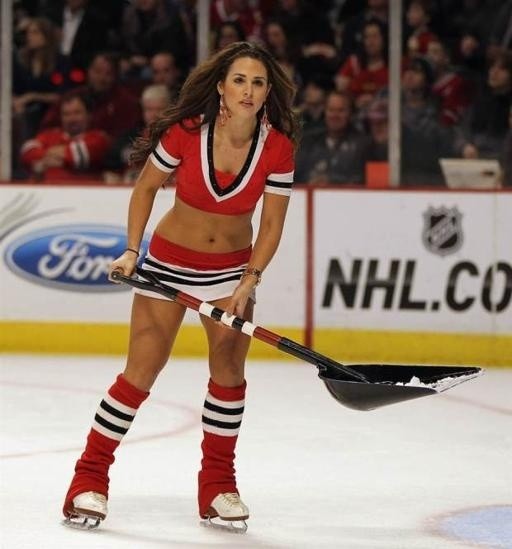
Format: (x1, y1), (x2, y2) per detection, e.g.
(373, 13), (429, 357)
(125, 247), (139, 258)
(241, 267), (261, 287)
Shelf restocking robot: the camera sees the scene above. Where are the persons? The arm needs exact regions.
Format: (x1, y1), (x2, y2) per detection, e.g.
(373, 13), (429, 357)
(10, 1), (512, 187)
(62, 39), (302, 522)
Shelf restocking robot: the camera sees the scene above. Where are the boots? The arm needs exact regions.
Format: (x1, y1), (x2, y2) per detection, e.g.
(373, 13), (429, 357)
(63, 374), (149, 519)
(199, 378), (249, 521)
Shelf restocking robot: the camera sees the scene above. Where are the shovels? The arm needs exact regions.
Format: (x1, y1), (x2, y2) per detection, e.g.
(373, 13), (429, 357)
(111, 267), (483, 412)
